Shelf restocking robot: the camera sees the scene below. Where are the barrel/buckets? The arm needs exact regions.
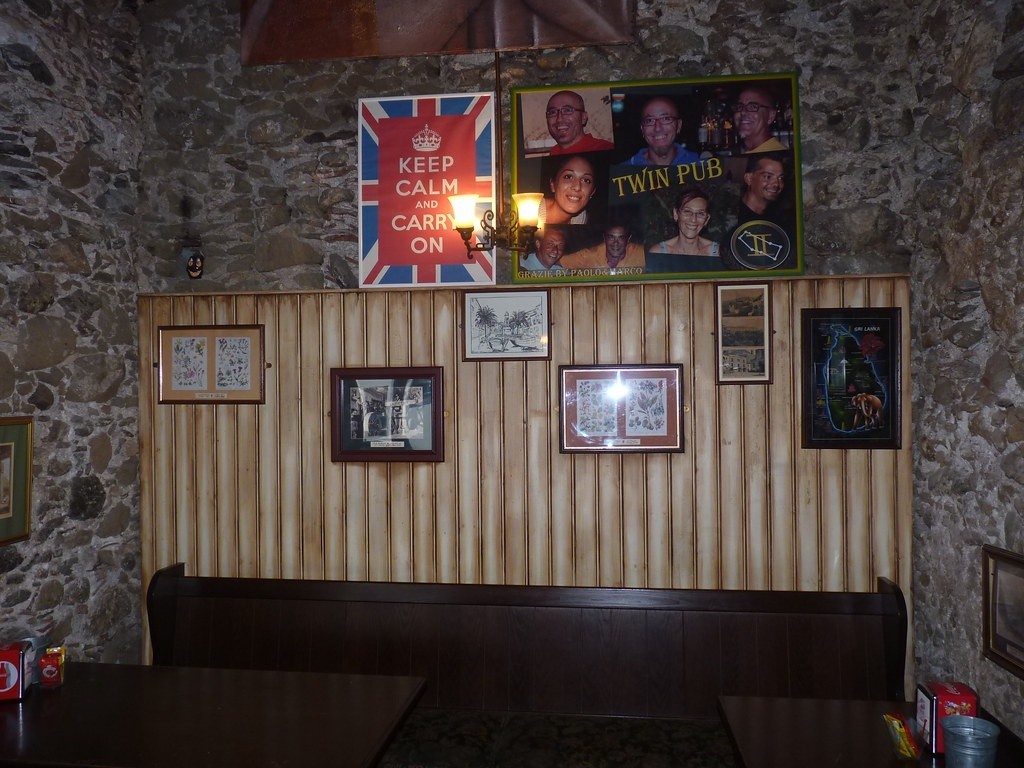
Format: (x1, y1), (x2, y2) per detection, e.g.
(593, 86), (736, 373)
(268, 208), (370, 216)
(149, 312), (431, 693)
(941, 715), (1000, 768)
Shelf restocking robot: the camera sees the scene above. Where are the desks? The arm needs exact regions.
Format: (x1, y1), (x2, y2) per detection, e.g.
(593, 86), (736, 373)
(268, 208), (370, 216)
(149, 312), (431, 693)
(0, 662), (427, 768)
(715, 696), (1024, 768)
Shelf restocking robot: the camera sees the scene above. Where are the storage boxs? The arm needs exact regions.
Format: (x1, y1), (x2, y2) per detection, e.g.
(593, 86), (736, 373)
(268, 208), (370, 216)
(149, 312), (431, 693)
(40, 647), (66, 687)
(0, 640), (33, 702)
(914, 681), (981, 756)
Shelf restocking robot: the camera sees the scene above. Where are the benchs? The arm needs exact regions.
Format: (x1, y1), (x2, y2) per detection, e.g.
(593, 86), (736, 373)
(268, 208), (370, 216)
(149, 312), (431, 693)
(146, 562), (908, 768)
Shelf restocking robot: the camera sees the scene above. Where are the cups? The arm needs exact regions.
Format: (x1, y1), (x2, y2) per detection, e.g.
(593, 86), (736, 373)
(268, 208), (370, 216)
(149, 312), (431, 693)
(21, 636), (53, 685)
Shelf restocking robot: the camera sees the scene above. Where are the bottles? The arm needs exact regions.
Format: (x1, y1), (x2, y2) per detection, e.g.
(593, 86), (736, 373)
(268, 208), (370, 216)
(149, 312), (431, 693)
(697, 108), (734, 151)
(774, 102), (793, 130)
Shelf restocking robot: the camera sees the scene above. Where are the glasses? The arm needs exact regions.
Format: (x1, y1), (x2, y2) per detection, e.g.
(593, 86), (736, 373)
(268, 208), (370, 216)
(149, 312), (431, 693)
(731, 102), (774, 115)
(641, 115), (682, 126)
(546, 105), (588, 117)
(676, 206), (709, 219)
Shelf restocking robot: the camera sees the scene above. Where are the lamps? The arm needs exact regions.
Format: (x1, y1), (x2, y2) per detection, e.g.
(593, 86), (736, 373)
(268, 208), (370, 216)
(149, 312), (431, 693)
(448, 192), (543, 262)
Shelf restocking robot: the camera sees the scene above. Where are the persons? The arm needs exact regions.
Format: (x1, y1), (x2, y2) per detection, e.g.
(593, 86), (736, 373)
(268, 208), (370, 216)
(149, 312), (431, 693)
(650, 188), (721, 257)
(546, 156), (598, 224)
(523, 217), (645, 269)
(733, 157), (785, 215)
(545, 91), (615, 156)
(519, 228), (566, 276)
(625, 94), (711, 166)
(734, 85), (789, 153)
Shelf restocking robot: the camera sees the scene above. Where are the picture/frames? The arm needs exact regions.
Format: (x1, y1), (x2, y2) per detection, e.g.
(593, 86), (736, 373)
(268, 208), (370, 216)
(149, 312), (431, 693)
(800, 307), (904, 450)
(981, 544), (1024, 681)
(357, 92), (497, 288)
(461, 288), (552, 362)
(330, 366), (445, 464)
(509, 72), (804, 283)
(713, 280), (774, 384)
(157, 324), (266, 405)
(557, 363), (685, 454)
(0, 415), (34, 547)
